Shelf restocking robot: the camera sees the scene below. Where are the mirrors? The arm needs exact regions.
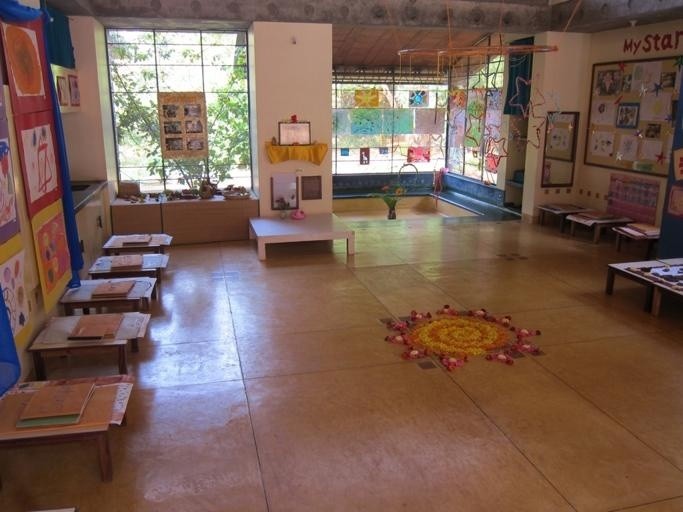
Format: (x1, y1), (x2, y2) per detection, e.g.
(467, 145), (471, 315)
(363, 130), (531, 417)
(541, 112), (579, 188)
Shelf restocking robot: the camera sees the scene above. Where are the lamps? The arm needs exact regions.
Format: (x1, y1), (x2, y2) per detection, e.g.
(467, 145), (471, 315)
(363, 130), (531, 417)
(380, 0), (586, 57)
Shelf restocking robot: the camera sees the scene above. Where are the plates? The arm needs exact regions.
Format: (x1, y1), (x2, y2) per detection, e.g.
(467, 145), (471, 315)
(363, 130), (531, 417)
(225, 190), (251, 199)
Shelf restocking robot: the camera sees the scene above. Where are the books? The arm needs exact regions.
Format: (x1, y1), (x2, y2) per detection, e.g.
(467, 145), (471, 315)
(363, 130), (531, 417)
(14, 235), (152, 429)
(578, 211), (660, 241)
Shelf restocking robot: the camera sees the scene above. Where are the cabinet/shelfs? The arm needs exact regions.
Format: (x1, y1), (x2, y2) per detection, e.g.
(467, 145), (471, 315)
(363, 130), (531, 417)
(111, 191), (260, 246)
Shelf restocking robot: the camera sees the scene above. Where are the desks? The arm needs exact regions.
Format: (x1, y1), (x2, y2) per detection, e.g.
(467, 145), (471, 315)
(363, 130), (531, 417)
(0, 372), (138, 483)
(102, 234), (174, 253)
(248, 211), (355, 261)
(536, 202), (661, 261)
(605, 257), (683, 318)
(88, 253), (173, 300)
(58, 277), (158, 316)
(25, 313), (152, 381)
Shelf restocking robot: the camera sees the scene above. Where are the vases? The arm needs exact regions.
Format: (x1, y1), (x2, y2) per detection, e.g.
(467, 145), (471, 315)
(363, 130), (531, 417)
(270, 175), (299, 210)
(280, 210), (286, 219)
(388, 208), (397, 220)
(278, 122), (312, 145)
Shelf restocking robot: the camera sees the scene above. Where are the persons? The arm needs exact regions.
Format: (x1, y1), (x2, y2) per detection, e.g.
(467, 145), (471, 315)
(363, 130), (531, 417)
(596, 71), (619, 96)
(618, 107), (637, 125)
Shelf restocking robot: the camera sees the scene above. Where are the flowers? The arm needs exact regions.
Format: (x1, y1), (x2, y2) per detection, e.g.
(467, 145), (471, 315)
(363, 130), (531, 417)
(382, 183), (405, 209)
(276, 194), (295, 211)
